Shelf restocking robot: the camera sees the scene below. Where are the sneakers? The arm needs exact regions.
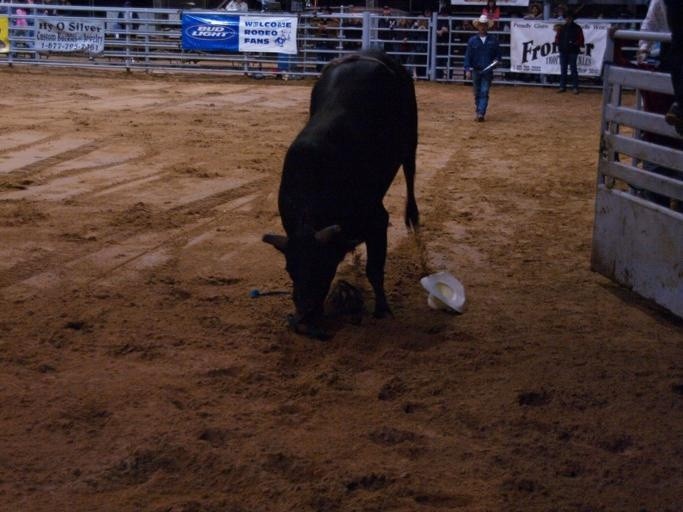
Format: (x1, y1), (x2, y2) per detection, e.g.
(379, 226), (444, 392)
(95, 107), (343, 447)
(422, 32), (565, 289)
(665, 103), (683, 137)
(573, 89), (578, 94)
(475, 113), (484, 121)
(556, 88), (566, 93)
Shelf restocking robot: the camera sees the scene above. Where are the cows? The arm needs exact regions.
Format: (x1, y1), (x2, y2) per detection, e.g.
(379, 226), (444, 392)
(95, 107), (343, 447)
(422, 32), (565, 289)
(261, 49), (417, 333)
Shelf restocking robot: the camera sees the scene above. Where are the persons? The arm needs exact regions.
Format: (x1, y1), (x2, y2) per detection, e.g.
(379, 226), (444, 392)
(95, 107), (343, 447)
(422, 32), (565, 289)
(555, 8), (584, 95)
(635, 0), (683, 208)
(182, 1), (565, 81)
(463, 15), (501, 121)
(1, 1), (156, 67)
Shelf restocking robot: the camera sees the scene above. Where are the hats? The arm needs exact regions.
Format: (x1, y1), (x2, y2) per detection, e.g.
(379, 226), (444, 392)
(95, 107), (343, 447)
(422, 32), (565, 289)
(420, 272), (465, 313)
(472, 15), (494, 29)
